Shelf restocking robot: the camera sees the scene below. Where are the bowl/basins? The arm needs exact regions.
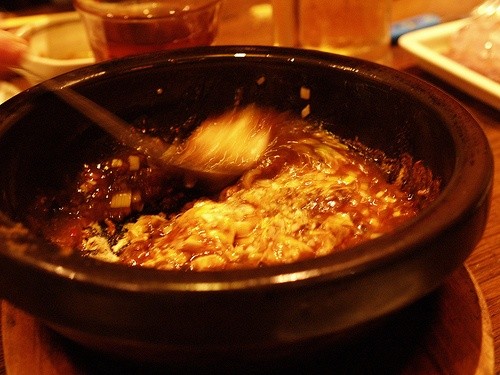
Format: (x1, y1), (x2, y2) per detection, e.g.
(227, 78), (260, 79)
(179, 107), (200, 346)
(1, 42), (493, 373)
(11, 13), (101, 79)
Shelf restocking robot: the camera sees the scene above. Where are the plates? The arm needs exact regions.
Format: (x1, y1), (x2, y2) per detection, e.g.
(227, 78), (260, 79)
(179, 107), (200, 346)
(400, 18), (500, 112)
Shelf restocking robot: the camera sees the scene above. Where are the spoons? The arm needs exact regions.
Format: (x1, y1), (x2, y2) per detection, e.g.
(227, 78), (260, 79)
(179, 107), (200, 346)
(1, 53), (248, 187)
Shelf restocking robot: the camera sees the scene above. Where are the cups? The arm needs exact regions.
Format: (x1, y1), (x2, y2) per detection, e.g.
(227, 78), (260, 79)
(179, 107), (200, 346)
(269, 1), (392, 66)
(73, 0), (221, 65)
